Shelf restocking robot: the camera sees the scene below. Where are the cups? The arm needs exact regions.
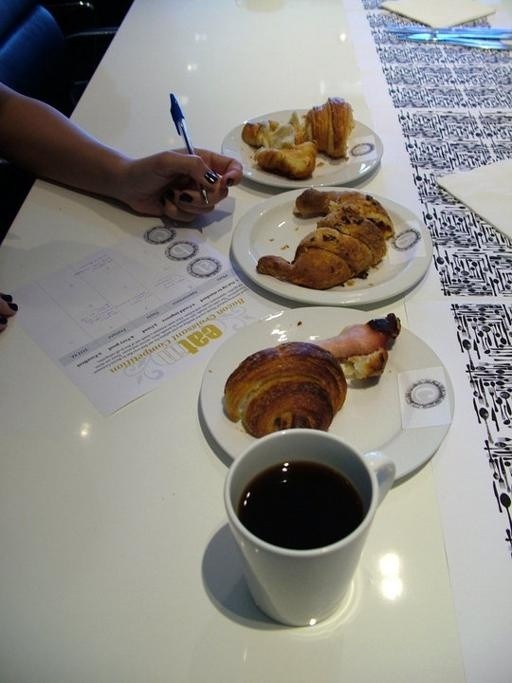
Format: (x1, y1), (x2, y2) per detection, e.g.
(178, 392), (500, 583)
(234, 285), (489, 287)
(223, 428), (395, 625)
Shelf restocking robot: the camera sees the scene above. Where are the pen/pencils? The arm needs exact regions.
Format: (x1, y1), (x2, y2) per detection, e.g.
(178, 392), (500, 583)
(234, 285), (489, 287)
(170, 93), (209, 205)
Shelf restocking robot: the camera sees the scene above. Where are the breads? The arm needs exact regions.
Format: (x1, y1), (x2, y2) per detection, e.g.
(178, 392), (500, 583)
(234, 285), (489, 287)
(239, 95), (354, 177)
(319, 313), (402, 383)
(221, 340), (349, 437)
(250, 189), (395, 292)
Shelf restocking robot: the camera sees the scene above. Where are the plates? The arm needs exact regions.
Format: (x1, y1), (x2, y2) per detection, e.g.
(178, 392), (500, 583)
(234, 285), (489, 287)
(199, 306), (454, 480)
(221, 109), (384, 188)
(231, 187), (433, 307)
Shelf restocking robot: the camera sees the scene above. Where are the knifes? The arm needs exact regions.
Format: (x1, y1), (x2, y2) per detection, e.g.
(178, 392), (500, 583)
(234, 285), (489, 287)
(383, 25), (512, 52)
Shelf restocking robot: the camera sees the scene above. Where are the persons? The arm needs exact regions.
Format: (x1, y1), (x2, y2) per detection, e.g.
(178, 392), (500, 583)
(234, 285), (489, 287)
(0, 79), (242, 330)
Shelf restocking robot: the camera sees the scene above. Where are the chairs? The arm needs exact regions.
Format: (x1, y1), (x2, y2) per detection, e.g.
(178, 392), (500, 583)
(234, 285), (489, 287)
(0, 0), (119, 118)
